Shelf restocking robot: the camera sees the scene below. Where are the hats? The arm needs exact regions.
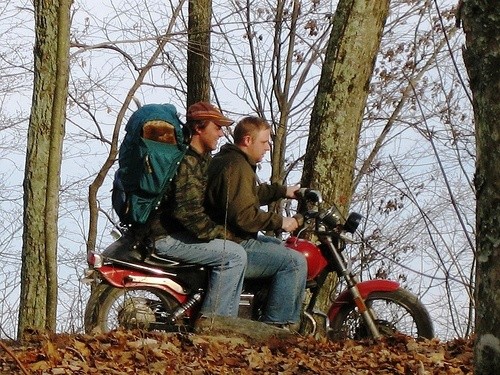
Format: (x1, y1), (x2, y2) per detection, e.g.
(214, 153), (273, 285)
(187, 101), (235, 126)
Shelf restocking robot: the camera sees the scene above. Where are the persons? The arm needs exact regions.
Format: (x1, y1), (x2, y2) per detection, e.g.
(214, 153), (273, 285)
(131, 101), (248, 333)
(204, 118), (308, 333)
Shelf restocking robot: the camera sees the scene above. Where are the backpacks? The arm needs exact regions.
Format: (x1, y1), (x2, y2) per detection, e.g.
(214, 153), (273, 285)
(111, 103), (192, 224)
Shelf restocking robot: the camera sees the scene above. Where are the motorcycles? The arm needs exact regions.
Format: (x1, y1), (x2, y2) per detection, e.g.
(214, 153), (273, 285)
(84, 188), (434, 340)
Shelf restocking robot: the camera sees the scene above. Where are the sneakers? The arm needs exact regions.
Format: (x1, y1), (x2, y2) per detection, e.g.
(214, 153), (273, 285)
(194, 314), (206, 333)
